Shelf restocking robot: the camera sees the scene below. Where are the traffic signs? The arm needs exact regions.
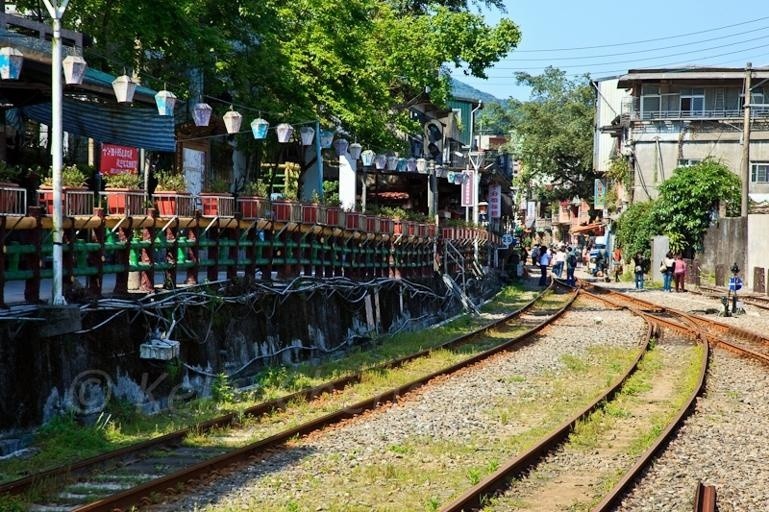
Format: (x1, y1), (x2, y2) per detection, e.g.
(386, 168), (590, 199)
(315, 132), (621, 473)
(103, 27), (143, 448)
(501, 234), (513, 246)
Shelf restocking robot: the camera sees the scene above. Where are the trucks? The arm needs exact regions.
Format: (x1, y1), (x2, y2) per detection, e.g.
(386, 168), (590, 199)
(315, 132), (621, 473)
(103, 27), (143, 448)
(588, 235), (606, 259)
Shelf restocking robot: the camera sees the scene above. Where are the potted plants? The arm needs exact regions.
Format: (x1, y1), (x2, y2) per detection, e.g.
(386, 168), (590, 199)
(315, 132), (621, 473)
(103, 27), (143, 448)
(302, 189), (319, 224)
(199, 169), (233, 217)
(380, 206), (478, 239)
(103, 167), (145, 217)
(326, 197), (342, 226)
(237, 180), (269, 220)
(275, 191), (292, 220)
(152, 166), (191, 218)
(366, 208), (376, 233)
(38, 163), (89, 214)
(0, 158), (33, 213)
(345, 204), (360, 229)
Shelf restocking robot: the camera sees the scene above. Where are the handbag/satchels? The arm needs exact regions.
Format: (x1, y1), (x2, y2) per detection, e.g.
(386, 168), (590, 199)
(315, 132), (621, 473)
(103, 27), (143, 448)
(659, 262), (667, 272)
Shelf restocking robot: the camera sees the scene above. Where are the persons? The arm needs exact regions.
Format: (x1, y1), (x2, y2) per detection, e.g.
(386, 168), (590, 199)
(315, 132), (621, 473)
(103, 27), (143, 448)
(613, 244), (621, 282)
(582, 240), (609, 277)
(532, 244), (577, 287)
(633, 252), (643, 289)
(673, 255), (687, 292)
(661, 253), (675, 292)
(506, 239), (528, 280)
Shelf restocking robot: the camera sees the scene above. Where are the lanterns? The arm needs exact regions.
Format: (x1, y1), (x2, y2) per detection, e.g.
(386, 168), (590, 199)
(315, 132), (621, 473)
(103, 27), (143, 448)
(362, 150), (469, 184)
(335, 141), (348, 155)
(278, 124), (294, 143)
(191, 103), (213, 127)
(320, 131), (333, 148)
(0, 47), (23, 80)
(111, 75), (137, 102)
(62, 56), (87, 85)
(350, 143), (362, 159)
(300, 127), (315, 146)
(223, 111), (242, 134)
(251, 119), (269, 139)
(155, 90), (177, 116)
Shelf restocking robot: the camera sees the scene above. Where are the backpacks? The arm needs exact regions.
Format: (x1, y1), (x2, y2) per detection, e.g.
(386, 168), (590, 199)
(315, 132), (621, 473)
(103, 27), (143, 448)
(635, 266), (642, 272)
(569, 254), (577, 267)
